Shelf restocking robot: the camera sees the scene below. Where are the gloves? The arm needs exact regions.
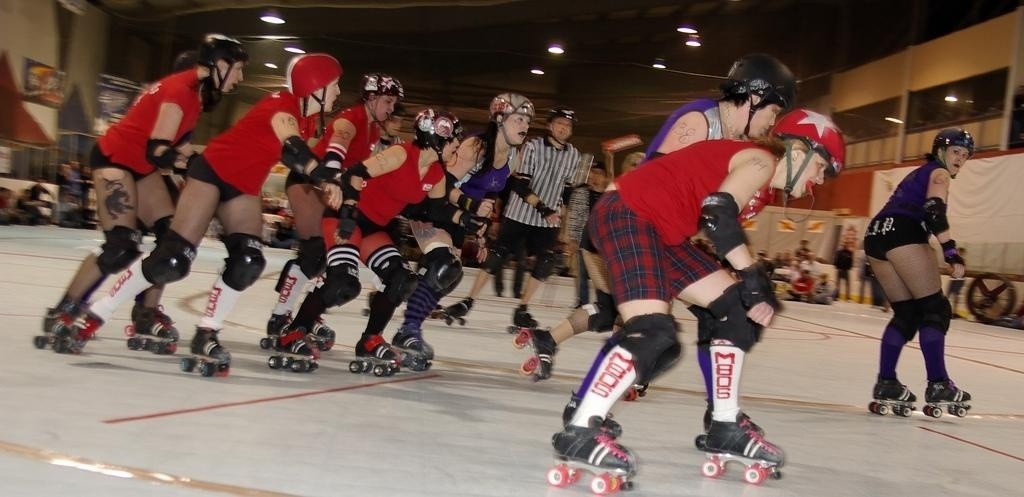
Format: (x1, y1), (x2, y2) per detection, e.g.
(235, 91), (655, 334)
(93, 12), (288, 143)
(457, 194), (484, 214)
(458, 212), (492, 235)
(738, 261), (781, 314)
(310, 162), (345, 189)
(940, 239), (966, 266)
(535, 200), (556, 218)
(337, 203), (358, 240)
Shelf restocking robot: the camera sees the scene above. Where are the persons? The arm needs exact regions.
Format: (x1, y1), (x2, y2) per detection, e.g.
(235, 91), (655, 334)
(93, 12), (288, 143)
(268, 107), (488, 375)
(52, 53), (342, 376)
(944, 246), (966, 318)
(548, 107), (847, 494)
(754, 240), (888, 306)
(863, 126), (975, 420)
(259, 72), (403, 350)
(0, 159), (93, 230)
(390, 94), (536, 372)
(32, 33), (244, 354)
(430, 105), (581, 332)
(514, 51), (796, 451)
(368, 103), (405, 157)
(563, 161), (610, 309)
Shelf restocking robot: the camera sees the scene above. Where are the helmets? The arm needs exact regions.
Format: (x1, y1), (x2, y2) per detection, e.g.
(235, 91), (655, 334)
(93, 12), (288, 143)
(546, 105), (578, 128)
(285, 52), (341, 96)
(932, 125), (975, 156)
(728, 52), (798, 110)
(414, 108), (464, 145)
(489, 93), (536, 118)
(770, 107), (847, 179)
(197, 34), (250, 66)
(360, 71), (405, 99)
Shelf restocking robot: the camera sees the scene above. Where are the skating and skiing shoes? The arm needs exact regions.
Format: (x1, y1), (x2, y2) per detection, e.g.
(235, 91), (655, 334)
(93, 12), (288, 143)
(259, 313), (291, 351)
(35, 307), (81, 355)
(623, 382), (649, 401)
(349, 335), (400, 377)
(702, 414), (786, 485)
(432, 297), (473, 326)
(869, 376), (918, 416)
(79, 300), (105, 340)
(392, 331), (436, 372)
(701, 399), (764, 436)
(181, 325), (232, 379)
(310, 320), (335, 352)
(507, 305), (538, 336)
(561, 390), (623, 439)
(923, 379), (972, 417)
(268, 325), (320, 374)
(125, 296), (180, 355)
(546, 424), (638, 497)
(514, 326), (559, 382)
